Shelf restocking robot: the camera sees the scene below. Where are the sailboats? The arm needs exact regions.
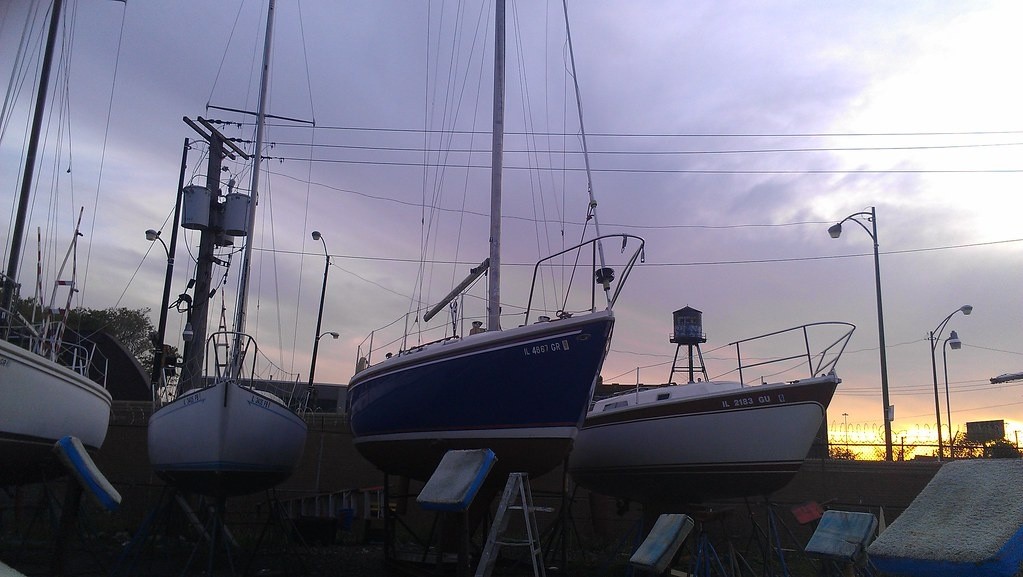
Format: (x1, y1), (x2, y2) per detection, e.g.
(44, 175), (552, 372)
(0, 1), (316, 494)
(344, 0), (647, 488)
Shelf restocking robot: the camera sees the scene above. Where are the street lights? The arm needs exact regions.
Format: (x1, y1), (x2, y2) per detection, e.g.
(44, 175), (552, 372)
(828, 205), (893, 460)
(145, 136), (226, 401)
(928, 305), (974, 461)
(305, 230), (339, 413)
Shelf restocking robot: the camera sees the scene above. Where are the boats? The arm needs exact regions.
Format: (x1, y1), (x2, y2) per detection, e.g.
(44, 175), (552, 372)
(570, 320), (857, 504)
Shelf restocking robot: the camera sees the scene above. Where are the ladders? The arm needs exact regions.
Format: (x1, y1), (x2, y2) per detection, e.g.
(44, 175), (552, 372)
(474, 469), (548, 577)
